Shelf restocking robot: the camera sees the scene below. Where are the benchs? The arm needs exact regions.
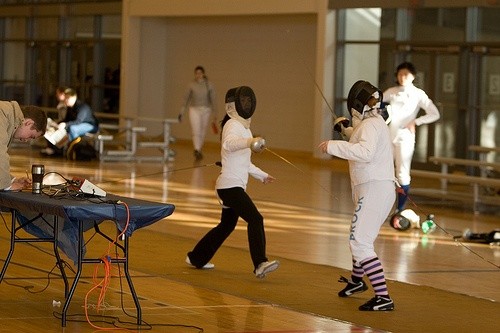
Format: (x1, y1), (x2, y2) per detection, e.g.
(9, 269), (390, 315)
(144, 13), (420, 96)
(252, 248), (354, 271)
(42, 109), (179, 162)
(408, 145), (500, 215)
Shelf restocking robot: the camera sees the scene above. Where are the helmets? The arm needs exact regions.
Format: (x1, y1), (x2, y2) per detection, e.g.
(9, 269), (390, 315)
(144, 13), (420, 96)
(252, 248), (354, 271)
(224, 87), (257, 129)
(346, 79), (383, 119)
(390, 208), (421, 232)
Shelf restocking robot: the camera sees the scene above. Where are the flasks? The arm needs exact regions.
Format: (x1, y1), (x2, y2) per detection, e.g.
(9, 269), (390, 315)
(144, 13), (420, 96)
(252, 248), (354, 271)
(32, 164), (45, 195)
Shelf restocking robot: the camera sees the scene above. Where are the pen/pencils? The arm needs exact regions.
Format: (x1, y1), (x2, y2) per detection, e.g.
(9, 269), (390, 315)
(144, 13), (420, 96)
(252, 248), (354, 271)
(24, 168), (31, 182)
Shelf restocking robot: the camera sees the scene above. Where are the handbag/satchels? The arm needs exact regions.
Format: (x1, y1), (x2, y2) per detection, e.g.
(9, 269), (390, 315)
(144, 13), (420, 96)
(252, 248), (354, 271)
(212, 117), (219, 135)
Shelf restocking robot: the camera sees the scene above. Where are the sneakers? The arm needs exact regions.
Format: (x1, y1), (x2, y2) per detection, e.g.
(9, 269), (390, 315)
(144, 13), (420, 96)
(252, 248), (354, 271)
(253, 258), (279, 279)
(183, 254), (215, 269)
(359, 293), (395, 313)
(337, 274), (369, 297)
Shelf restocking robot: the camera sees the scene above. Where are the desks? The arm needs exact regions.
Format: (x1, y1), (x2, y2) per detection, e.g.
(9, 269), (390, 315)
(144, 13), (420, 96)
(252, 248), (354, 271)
(0, 191), (175, 333)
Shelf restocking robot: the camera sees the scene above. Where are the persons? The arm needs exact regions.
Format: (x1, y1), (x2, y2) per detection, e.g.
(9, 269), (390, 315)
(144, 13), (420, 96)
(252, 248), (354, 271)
(177, 66), (218, 161)
(39, 84), (98, 159)
(185, 86), (280, 279)
(380, 61), (440, 214)
(317, 79), (398, 312)
(0, 99), (47, 193)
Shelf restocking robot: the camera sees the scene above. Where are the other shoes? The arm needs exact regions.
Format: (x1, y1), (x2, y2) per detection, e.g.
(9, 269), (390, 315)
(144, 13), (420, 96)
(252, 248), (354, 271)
(40, 148), (56, 157)
(194, 148), (202, 163)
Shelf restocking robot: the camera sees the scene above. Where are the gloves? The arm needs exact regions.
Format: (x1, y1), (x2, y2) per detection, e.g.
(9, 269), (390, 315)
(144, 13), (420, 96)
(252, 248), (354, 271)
(177, 114), (182, 124)
